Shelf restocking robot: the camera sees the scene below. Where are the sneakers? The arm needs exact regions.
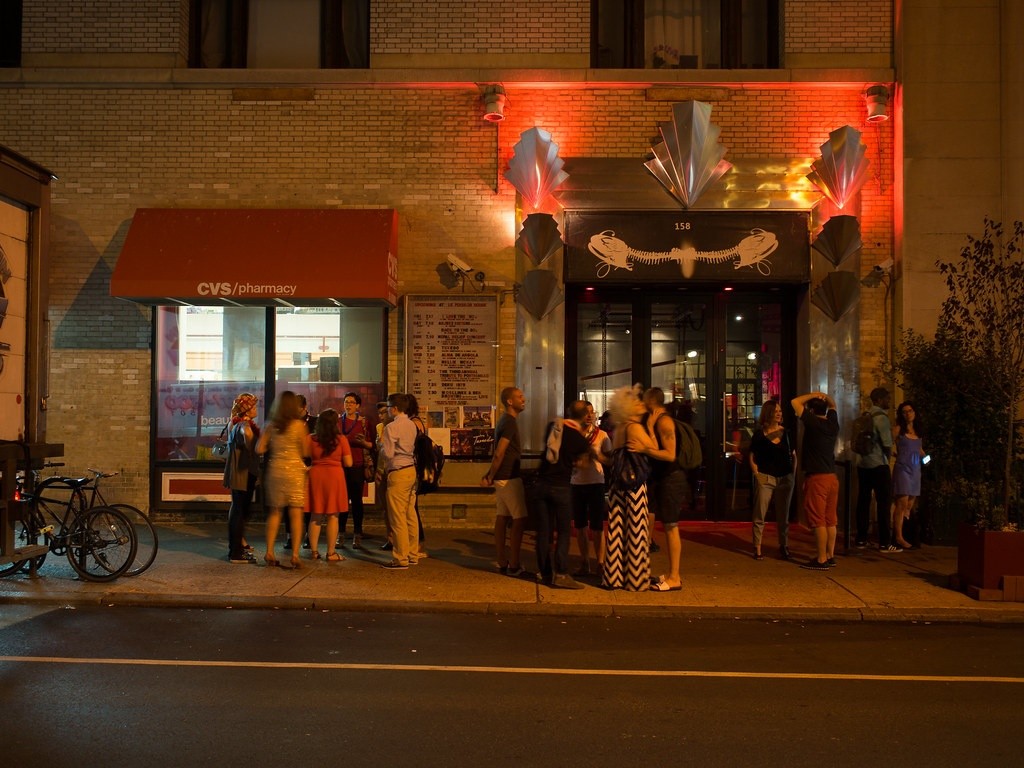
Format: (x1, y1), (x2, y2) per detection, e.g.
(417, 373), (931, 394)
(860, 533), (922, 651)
(381, 560), (418, 569)
(228, 549), (256, 564)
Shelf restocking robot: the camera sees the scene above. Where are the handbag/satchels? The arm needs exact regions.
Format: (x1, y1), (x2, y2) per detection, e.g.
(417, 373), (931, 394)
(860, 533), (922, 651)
(221, 422), (249, 491)
(212, 440), (229, 459)
(609, 423), (652, 490)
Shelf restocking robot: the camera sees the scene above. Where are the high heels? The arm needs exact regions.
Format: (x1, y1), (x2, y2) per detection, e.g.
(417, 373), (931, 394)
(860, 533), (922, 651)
(290, 556), (305, 570)
(264, 553), (280, 568)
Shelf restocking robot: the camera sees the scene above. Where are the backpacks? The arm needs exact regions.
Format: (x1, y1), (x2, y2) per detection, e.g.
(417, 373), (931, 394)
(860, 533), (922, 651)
(654, 412), (702, 469)
(850, 411), (889, 455)
(413, 422), (445, 493)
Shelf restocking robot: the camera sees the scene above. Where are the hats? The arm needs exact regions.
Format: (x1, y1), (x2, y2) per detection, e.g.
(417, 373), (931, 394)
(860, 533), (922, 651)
(234, 393), (258, 414)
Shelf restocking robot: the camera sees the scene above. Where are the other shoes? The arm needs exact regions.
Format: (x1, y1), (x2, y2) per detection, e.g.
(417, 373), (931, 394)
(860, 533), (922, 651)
(335, 535), (345, 548)
(303, 538), (310, 548)
(352, 536), (361, 548)
(283, 538), (291, 549)
(379, 540), (394, 550)
(500, 528), (919, 593)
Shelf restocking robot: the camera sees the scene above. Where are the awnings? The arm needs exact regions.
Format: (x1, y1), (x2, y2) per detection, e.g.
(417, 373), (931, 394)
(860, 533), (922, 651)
(109, 208), (399, 307)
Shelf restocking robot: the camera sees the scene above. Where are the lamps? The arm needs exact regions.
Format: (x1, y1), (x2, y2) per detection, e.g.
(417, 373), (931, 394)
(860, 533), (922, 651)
(482, 84), (506, 123)
(866, 84), (891, 123)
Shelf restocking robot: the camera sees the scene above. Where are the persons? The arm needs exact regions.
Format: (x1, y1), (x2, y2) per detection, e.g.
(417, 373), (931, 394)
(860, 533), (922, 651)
(227, 392), (261, 564)
(571, 402), (613, 577)
(256, 391), (311, 569)
(855, 388), (903, 553)
(284, 394), (319, 550)
(536, 401), (607, 589)
(790, 392), (840, 570)
(481, 387), (535, 579)
(890, 401), (930, 548)
(306, 408), (353, 561)
(600, 389), (662, 592)
(335, 392), (373, 549)
(748, 401), (797, 561)
(626, 387), (685, 592)
(374, 392), (426, 569)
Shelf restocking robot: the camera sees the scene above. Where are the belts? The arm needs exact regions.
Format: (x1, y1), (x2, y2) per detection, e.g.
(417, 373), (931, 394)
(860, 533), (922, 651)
(388, 464), (414, 473)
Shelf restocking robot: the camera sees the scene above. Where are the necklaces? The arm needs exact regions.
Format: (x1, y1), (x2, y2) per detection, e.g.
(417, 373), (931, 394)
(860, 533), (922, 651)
(342, 412), (359, 435)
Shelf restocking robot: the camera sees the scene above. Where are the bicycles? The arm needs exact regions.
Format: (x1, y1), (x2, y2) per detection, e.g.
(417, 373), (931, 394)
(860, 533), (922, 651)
(0, 466), (159, 583)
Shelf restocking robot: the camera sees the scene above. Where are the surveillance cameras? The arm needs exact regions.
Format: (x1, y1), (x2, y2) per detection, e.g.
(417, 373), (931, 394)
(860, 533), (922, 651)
(873, 256), (895, 273)
(447, 254), (474, 273)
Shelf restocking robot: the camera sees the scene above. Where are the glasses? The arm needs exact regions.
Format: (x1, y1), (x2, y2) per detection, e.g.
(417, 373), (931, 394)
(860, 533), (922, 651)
(378, 410), (387, 415)
(344, 400), (356, 405)
(298, 409), (304, 416)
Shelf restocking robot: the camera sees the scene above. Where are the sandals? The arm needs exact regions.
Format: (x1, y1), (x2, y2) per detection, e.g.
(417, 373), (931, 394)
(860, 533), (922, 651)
(311, 551), (320, 560)
(326, 552), (346, 561)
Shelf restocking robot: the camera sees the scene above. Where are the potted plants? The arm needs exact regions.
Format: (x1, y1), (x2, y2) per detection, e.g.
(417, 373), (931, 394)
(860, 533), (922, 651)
(934, 214), (1024, 600)
